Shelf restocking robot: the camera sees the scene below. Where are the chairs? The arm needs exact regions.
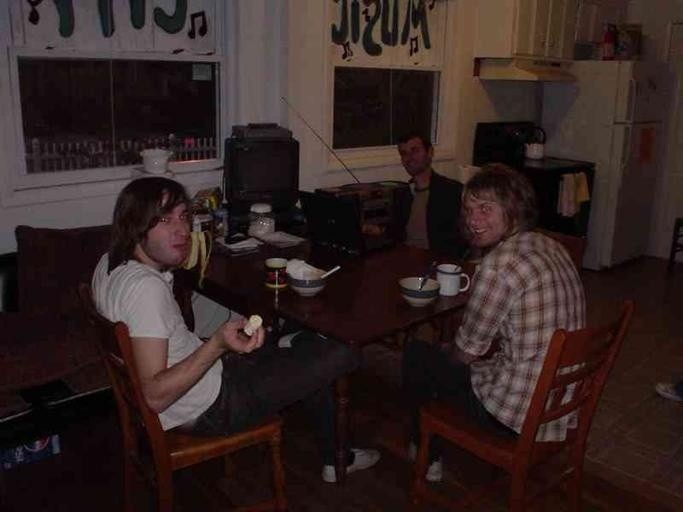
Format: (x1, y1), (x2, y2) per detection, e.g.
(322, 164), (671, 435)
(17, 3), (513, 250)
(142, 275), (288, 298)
(408, 298), (633, 512)
(78, 280), (289, 512)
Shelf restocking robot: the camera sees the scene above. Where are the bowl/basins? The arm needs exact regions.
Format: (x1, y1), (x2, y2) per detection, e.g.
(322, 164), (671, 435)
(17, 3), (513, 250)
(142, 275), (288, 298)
(288, 270), (328, 296)
(399, 277), (440, 308)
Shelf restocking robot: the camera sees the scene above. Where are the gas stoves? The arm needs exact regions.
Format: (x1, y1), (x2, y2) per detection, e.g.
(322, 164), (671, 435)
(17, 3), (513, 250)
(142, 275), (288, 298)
(475, 154), (597, 170)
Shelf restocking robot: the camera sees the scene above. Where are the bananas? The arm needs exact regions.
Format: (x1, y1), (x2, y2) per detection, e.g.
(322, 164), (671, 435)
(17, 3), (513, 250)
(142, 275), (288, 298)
(244, 315), (263, 336)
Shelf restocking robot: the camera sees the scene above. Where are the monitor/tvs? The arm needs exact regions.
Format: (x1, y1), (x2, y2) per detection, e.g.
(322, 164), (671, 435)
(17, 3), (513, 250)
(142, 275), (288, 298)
(224, 139), (299, 200)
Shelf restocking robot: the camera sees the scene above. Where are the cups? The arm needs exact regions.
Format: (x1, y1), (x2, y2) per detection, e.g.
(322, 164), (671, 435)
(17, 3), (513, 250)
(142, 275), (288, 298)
(140, 149), (172, 174)
(436, 263), (470, 298)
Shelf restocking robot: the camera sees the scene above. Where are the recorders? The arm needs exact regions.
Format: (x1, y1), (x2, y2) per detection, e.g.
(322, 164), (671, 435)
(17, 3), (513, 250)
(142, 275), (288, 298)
(340, 183), (394, 210)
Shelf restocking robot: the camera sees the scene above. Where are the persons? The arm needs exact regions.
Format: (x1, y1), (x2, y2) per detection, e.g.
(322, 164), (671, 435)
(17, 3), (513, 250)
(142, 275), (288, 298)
(401, 162), (588, 484)
(88, 177), (381, 483)
(383, 131), (465, 258)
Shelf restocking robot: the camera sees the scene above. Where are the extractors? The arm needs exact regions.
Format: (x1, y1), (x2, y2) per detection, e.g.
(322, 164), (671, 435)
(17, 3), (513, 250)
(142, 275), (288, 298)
(478, 58), (579, 82)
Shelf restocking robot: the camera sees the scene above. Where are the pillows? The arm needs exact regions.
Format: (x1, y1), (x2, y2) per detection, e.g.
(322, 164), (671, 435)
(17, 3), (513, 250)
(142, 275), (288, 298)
(15, 225), (119, 313)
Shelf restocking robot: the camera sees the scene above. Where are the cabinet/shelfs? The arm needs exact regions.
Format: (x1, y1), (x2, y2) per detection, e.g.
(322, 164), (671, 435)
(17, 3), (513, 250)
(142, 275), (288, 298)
(474, 0), (619, 59)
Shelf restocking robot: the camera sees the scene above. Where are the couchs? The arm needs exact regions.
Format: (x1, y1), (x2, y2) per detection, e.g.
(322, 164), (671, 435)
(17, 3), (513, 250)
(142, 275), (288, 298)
(0, 252), (114, 449)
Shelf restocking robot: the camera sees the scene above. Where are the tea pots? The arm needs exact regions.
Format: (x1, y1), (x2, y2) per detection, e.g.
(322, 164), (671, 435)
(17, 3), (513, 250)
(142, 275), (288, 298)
(522, 126), (547, 160)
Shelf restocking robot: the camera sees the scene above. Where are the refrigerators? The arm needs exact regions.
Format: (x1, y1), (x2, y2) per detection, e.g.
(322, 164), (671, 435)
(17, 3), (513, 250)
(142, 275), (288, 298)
(542, 60), (670, 272)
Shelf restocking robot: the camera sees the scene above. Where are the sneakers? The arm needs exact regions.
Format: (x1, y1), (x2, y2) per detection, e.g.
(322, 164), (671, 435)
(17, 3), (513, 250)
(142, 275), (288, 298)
(323, 447), (380, 483)
(409, 439), (443, 481)
(655, 382), (681, 401)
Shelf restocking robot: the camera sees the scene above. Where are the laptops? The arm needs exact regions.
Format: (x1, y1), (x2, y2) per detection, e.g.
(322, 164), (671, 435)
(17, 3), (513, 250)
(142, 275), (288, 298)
(300, 191), (395, 258)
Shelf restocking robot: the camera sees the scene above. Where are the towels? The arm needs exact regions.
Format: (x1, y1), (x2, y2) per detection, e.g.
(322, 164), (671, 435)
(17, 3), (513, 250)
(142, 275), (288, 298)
(573, 173), (591, 206)
(558, 174), (582, 219)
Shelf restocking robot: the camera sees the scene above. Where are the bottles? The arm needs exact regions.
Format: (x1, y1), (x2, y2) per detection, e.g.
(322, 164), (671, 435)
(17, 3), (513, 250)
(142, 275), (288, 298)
(248, 203), (275, 235)
(604, 26), (616, 61)
